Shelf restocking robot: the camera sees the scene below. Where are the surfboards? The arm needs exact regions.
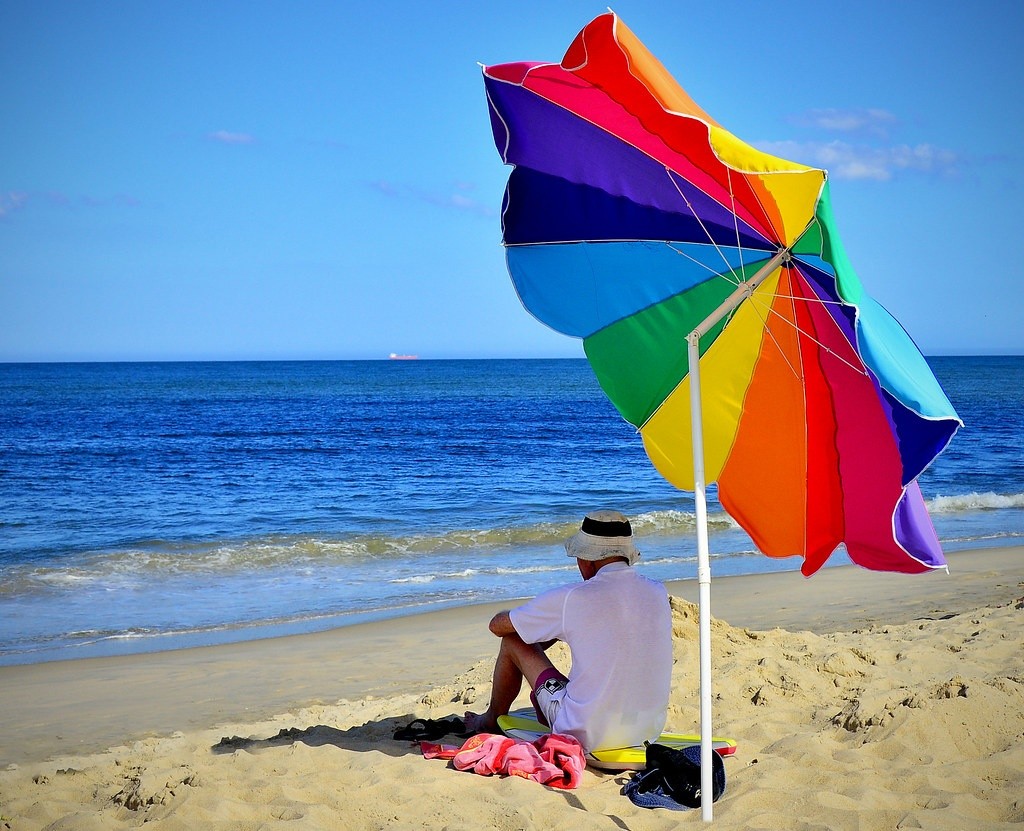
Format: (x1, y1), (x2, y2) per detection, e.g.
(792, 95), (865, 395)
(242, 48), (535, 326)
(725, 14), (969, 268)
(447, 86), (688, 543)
(498, 705), (738, 771)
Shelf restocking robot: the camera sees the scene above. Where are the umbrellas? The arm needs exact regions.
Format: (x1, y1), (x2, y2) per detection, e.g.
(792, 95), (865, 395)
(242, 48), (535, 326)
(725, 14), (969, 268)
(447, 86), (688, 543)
(477, 7), (965, 821)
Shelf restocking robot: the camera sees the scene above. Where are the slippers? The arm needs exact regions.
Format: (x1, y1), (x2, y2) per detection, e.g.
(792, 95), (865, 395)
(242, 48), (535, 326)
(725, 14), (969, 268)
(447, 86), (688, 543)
(393, 719), (444, 741)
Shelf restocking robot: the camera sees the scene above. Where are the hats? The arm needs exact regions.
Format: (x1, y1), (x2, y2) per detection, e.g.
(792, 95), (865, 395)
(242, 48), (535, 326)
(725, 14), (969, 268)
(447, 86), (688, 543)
(620, 739), (724, 811)
(565, 509), (641, 566)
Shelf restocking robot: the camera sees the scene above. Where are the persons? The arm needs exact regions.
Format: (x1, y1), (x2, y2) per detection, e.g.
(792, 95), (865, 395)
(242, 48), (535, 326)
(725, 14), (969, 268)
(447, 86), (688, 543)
(464, 511), (673, 753)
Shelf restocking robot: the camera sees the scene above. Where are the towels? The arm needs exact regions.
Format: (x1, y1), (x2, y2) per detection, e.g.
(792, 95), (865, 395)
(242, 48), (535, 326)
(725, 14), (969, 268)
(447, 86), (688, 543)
(419, 733), (586, 789)
